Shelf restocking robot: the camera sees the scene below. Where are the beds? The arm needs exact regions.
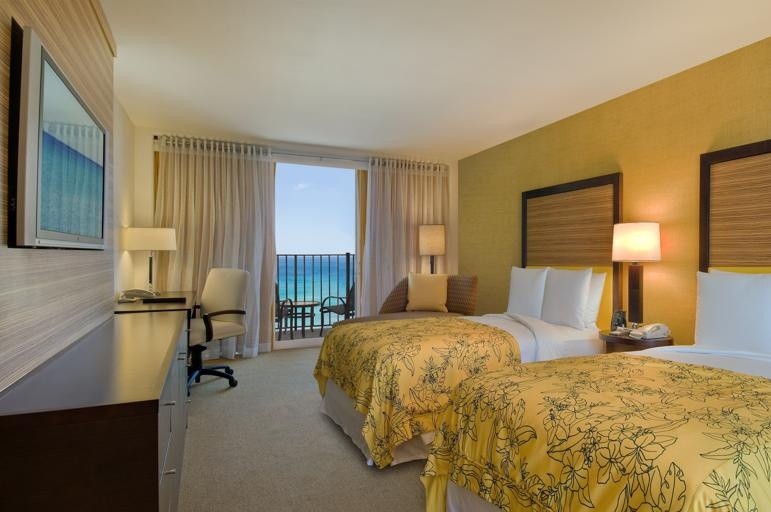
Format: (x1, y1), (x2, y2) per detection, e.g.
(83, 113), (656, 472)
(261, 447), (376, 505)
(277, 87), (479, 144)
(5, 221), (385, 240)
(420, 271), (771, 512)
(314, 263), (616, 470)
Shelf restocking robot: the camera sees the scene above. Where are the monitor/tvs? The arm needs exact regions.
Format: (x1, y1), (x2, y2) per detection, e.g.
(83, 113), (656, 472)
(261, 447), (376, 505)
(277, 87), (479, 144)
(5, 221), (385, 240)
(16, 27), (110, 251)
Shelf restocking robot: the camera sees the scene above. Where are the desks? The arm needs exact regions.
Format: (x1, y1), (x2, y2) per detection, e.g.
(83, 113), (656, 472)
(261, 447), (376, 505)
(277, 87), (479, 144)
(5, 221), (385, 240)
(114, 289), (198, 319)
(279, 301), (321, 337)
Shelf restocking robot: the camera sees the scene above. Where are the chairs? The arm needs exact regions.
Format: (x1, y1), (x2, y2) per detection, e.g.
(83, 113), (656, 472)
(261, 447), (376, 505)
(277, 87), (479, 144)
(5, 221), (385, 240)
(275, 283), (295, 341)
(186, 267), (252, 396)
(319, 282), (356, 336)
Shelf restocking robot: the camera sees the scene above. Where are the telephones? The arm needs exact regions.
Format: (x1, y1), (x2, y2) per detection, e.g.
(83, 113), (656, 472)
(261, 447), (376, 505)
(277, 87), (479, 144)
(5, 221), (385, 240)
(125, 289), (155, 299)
(629, 323), (671, 340)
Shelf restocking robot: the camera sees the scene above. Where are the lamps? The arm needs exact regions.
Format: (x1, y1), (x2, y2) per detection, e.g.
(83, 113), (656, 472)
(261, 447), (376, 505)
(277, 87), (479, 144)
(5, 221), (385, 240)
(123, 227), (178, 294)
(611, 220), (665, 331)
(419, 224), (447, 274)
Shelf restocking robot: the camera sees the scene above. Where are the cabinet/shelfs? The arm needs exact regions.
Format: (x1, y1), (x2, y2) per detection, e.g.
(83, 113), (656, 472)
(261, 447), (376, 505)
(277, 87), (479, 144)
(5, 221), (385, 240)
(0, 310), (190, 511)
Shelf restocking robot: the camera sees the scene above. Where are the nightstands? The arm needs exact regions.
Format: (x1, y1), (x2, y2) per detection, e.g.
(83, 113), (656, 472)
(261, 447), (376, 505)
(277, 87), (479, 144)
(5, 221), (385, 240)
(600, 331), (674, 354)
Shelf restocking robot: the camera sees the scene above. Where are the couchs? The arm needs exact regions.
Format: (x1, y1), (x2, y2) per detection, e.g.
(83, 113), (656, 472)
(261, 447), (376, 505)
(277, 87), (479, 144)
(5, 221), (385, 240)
(332, 274), (480, 329)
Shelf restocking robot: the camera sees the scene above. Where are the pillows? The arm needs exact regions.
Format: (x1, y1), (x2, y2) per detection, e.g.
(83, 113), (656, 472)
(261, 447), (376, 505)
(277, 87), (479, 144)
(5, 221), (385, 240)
(694, 271), (771, 356)
(405, 271), (451, 312)
(709, 268), (771, 279)
(507, 264), (608, 331)
